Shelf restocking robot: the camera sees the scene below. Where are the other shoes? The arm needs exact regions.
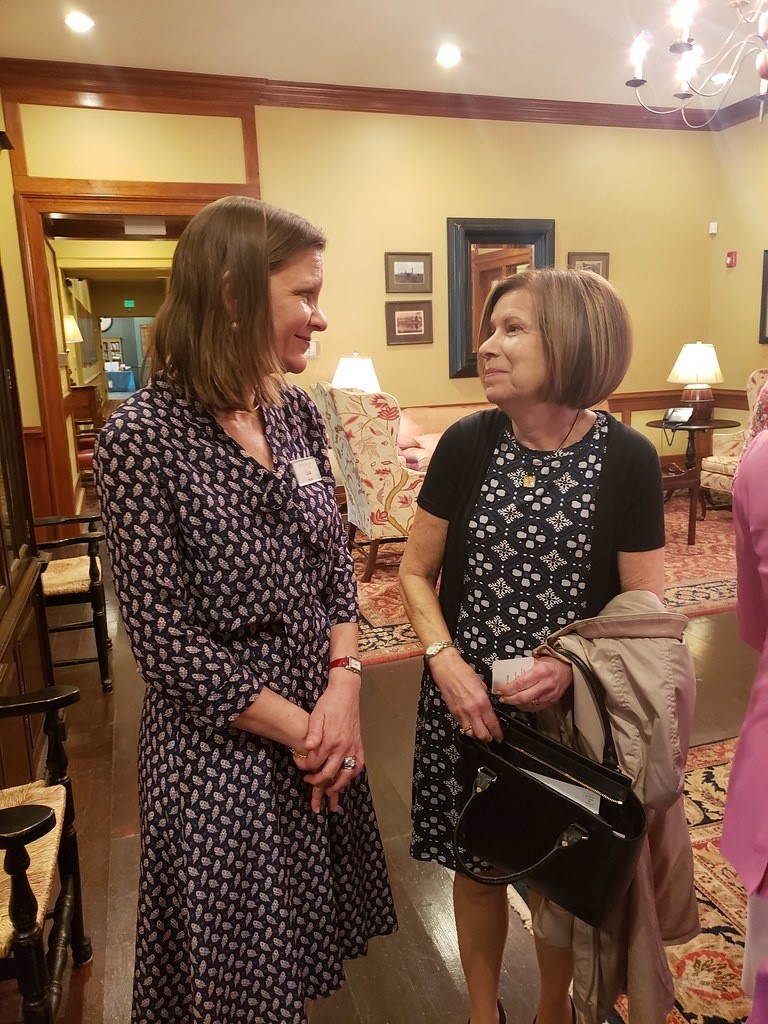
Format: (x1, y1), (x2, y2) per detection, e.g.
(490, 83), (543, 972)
(468, 999), (506, 1024)
(533, 994), (576, 1024)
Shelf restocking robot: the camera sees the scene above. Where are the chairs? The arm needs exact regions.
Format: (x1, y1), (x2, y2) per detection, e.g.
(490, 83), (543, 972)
(688, 368), (768, 545)
(73, 419), (108, 487)
(0, 684), (95, 1024)
(309, 381), (430, 583)
(32, 513), (114, 693)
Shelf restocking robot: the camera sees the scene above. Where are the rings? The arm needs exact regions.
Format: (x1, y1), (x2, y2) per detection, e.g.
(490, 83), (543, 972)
(343, 756), (356, 770)
(459, 724), (473, 734)
(531, 699), (539, 706)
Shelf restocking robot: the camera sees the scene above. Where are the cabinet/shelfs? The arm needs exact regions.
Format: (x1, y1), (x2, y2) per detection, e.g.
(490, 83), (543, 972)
(0, 551), (66, 790)
(101, 337), (123, 372)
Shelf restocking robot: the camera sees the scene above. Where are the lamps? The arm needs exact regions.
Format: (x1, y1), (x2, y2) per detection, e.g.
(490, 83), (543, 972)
(666, 341), (725, 425)
(61, 314), (84, 387)
(625, 0), (768, 129)
(330, 358), (381, 393)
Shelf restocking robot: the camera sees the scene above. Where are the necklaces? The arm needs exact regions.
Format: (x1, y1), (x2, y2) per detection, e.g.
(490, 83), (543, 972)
(233, 404), (261, 414)
(508, 408), (581, 489)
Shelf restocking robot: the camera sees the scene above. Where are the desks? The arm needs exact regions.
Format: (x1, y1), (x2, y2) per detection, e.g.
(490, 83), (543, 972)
(646, 419), (740, 501)
(105, 371), (135, 392)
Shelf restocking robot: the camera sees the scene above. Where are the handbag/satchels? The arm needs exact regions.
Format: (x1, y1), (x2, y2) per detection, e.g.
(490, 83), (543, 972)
(453, 708), (647, 929)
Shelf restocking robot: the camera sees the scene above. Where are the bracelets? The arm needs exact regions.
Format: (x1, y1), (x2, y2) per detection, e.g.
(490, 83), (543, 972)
(287, 746), (309, 760)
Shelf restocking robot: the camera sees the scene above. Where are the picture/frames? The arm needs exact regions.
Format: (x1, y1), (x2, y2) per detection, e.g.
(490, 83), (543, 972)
(568, 252), (610, 284)
(386, 300), (434, 346)
(446, 216), (555, 378)
(759, 249), (768, 343)
(384, 251), (433, 293)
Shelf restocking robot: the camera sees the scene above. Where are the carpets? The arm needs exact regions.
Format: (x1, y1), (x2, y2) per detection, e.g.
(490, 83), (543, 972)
(505, 734), (752, 1024)
(330, 484), (739, 665)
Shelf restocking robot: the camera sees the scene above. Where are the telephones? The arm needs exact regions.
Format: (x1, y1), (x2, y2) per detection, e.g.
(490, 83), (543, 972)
(663, 407), (694, 425)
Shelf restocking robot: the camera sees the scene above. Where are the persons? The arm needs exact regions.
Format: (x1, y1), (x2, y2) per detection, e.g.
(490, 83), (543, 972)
(397, 267), (703, 1024)
(91, 193), (402, 1024)
(719, 364), (768, 1024)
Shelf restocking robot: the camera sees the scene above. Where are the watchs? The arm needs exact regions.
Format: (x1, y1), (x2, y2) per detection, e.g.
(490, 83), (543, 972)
(329, 655), (363, 676)
(423, 639), (461, 661)
